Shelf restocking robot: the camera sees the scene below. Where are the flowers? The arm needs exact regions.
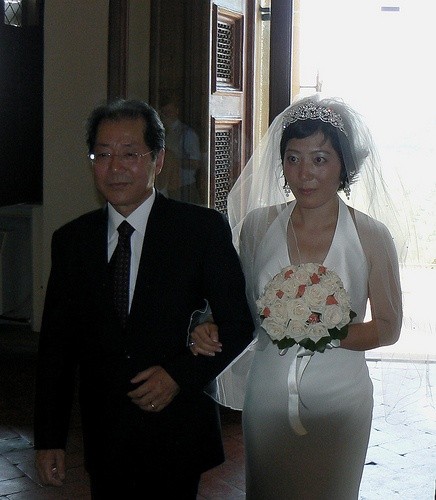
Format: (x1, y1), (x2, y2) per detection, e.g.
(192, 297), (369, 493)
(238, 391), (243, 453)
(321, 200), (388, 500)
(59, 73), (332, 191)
(256, 263), (357, 357)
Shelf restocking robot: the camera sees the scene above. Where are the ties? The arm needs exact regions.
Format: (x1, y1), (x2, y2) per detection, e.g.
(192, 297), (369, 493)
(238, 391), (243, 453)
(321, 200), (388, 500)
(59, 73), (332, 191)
(108, 220), (136, 325)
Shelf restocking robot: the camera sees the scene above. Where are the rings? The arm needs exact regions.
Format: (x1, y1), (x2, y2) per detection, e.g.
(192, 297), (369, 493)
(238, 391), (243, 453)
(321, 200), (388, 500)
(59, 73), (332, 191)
(150, 403), (156, 409)
(189, 342), (196, 345)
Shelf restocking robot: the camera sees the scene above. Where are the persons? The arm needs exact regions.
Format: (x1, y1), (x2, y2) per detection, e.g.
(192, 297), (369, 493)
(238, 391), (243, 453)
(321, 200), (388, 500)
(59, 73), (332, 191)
(186, 97), (404, 500)
(33, 99), (254, 500)
(173, 102), (201, 204)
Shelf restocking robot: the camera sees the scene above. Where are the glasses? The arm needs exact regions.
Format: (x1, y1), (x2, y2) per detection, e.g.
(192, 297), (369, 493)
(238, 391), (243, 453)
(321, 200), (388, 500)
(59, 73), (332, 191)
(87, 150), (153, 163)
